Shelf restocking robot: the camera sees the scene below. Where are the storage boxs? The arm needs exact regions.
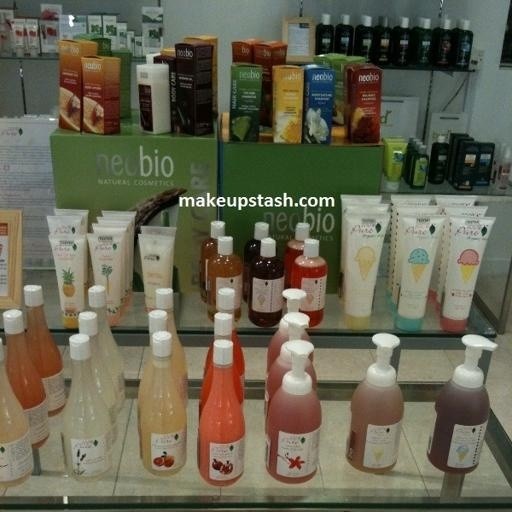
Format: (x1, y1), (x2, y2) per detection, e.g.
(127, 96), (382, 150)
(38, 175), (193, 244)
(49, 113), (219, 294)
(219, 114), (385, 295)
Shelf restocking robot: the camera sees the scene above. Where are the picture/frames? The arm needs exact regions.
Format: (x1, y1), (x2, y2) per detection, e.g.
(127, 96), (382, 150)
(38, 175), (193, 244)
(0, 208), (25, 310)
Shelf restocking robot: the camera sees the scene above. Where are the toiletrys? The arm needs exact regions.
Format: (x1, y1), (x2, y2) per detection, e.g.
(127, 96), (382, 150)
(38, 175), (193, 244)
(0, 4), (512, 487)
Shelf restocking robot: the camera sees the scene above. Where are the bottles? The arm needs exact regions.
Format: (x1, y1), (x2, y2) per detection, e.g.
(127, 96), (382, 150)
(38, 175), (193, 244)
(1, 283), (64, 486)
(198, 288), (247, 482)
(406, 131), (449, 189)
(139, 289), (190, 477)
(61, 285), (127, 480)
(198, 218), (331, 327)
(315, 12), (475, 68)
